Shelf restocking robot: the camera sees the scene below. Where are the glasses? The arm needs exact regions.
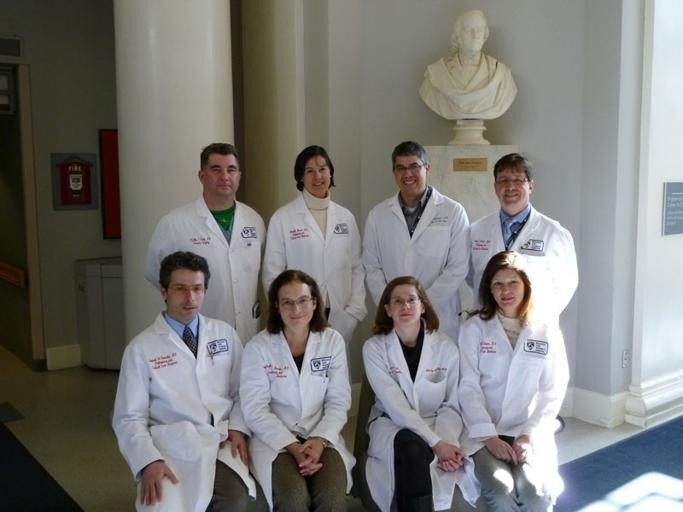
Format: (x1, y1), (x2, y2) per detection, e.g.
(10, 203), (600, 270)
(491, 178), (532, 193)
(393, 164), (427, 177)
(281, 296), (314, 310)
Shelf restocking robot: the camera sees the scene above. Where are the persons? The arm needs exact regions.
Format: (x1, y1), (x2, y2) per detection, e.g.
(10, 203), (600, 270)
(468, 153), (579, 322)
(360, 141), (470, 348)
(456, 250), (570, 512)
(110, 253), (254, 512)
(260, 143), (368, 351)
(144, 142), (268, 346)
(362, 281), (461, 512)
(415, 9), (521, 122)
(240, 273), (357, 511)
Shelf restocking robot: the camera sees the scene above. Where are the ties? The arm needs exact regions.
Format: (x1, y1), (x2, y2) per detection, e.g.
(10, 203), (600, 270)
(183, 327), (197, 357)
(502, 217), (517, 251)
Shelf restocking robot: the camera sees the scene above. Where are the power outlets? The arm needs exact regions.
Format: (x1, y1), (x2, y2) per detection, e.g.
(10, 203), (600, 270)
(623, 349), (632, 368)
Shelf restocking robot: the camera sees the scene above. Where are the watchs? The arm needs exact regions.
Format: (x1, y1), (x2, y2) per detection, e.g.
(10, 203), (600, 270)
(314, 437), (328, 449)
